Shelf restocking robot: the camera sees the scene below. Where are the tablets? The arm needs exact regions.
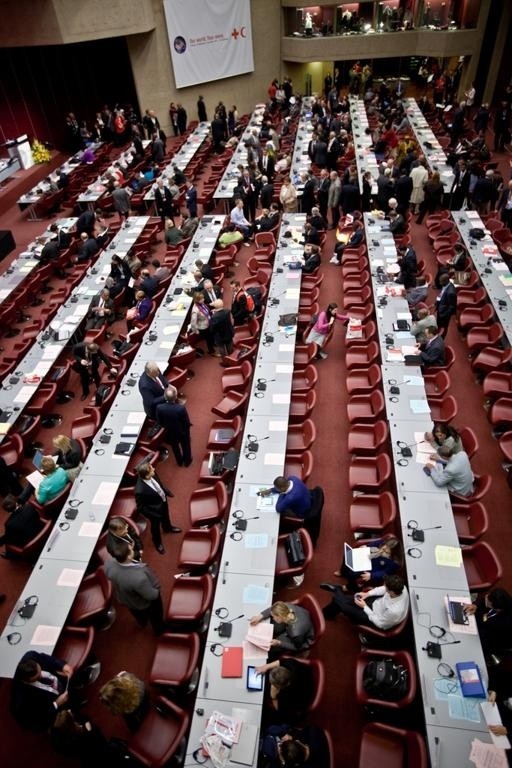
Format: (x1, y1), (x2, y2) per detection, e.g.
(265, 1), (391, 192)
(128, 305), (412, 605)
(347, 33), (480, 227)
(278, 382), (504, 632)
(247, 665), (264, 692)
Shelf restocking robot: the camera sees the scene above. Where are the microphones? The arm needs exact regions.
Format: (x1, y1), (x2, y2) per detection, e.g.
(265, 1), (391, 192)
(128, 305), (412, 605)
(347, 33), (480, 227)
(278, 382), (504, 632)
(412, 526), (442, 541)
(265, 330), (281, 342)
(258, 378), (276, 390)
(272, 290), (288, 304)
(427, 640), (463, 656)
(388, 379), (411, 394)
(215, 614), (245, 636)
(402, 440), (425, 456)
(236, 517), (260, 530)
(247, 435), (269, 451)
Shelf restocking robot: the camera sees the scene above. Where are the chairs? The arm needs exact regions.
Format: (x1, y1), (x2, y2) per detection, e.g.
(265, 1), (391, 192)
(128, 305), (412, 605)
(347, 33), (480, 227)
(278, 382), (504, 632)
(2, 64), (509, 766)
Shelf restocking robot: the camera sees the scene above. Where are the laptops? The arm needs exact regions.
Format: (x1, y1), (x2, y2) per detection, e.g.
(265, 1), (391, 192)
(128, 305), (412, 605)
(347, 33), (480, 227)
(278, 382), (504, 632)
(343, 541), (373, 573)
(47, 325), (70, 342)
(446, 594), (471, 625)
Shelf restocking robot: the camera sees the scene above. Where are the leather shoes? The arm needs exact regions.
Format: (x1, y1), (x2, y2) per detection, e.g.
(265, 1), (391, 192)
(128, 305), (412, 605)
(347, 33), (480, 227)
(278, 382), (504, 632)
(81, 392), (88, 401)
(156, 543), (165, 555)
(169, 527), (182, 533)
(319, 581), (343, 593)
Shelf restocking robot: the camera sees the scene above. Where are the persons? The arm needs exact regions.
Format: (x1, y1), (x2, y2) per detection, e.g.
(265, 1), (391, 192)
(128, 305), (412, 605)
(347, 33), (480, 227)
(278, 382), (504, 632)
(1, 0), (511, 768)
